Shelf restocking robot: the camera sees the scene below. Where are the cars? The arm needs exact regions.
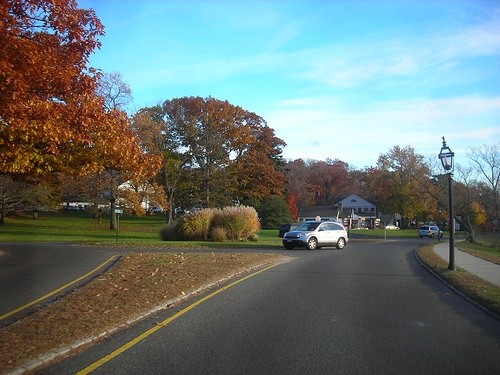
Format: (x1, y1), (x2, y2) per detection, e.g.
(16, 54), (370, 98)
(279, 223), (302, 238)
(146, 206), (161, 213)
(385, 224), (400, 231)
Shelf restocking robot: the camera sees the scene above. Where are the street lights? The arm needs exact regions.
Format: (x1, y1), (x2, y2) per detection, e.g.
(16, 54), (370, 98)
(437, 135), (455, 271)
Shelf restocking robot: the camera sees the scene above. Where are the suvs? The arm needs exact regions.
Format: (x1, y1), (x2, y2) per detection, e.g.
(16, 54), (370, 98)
(418, 226), (444, 239)
(283, 221), (349, 250)
